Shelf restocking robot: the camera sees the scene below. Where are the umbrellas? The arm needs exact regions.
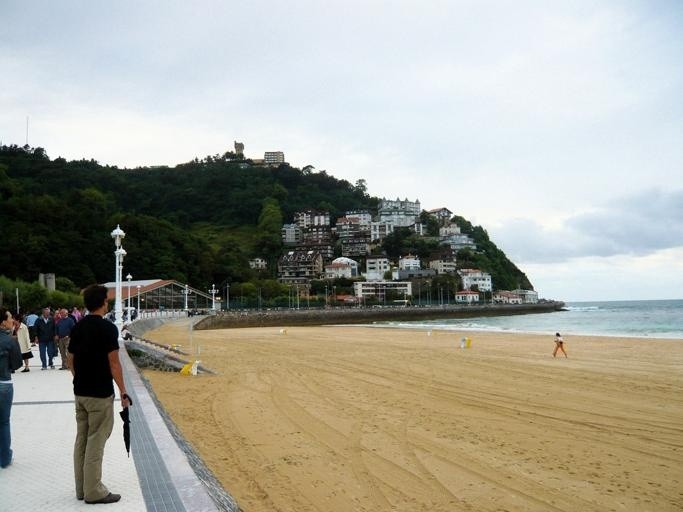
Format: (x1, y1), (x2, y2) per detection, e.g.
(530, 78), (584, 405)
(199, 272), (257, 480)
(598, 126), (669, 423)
(119, 393), (132, 457)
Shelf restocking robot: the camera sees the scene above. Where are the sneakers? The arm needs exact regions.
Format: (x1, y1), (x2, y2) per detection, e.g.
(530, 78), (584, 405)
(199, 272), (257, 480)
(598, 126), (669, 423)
(20, 365), (65, 372)
(1, 448), (12, 468)
(84, 492), (120, 503)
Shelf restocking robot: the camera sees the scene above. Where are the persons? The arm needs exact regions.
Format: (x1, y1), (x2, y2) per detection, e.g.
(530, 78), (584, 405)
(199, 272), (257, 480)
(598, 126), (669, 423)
(68, 284), (129, 504)
(0, 307), (24, 468)
(553, 333), (567, 358)
(0, 307), (89, 373)
(106, 309), (137, 323)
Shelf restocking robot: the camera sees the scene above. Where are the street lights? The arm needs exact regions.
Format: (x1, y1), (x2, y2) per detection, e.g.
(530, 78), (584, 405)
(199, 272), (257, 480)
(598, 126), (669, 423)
(111, 224), (141, 341)
(181, 285), (191, 309)
(209, 284), (219, 309)
